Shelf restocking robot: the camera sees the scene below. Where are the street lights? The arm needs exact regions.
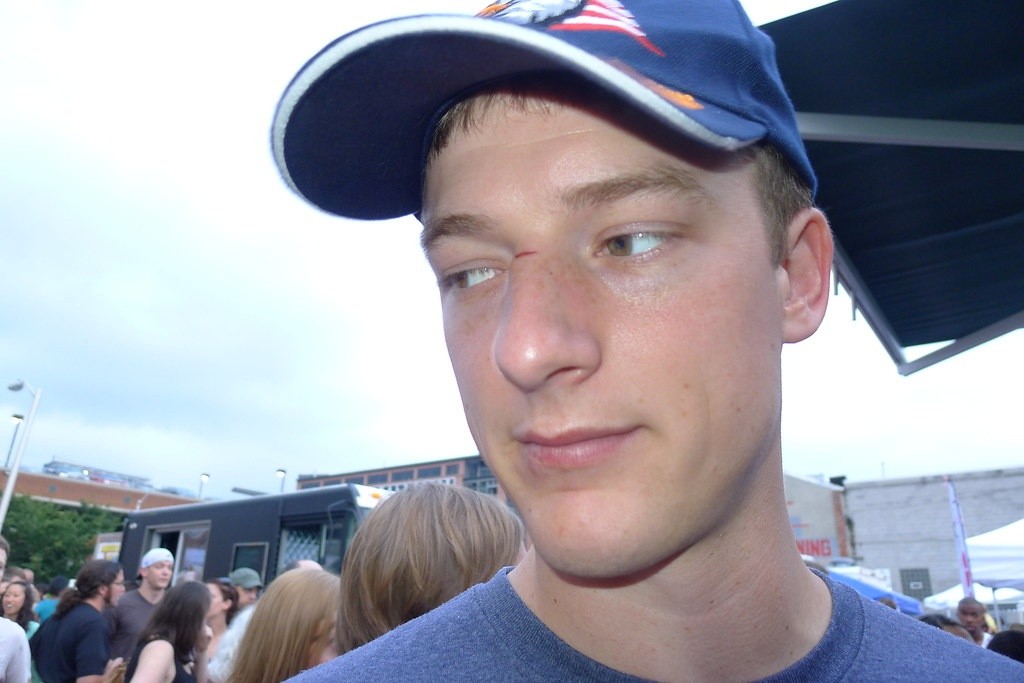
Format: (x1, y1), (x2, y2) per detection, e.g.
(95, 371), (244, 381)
(0, 378), (44, 536)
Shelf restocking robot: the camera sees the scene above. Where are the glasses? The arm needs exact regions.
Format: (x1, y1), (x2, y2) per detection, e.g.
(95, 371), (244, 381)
(113, 579), (127, 586)
(214, 576), (232, 588)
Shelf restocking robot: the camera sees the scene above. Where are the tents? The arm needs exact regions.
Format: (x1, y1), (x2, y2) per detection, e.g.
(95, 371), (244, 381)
(965, 519), (1024, 632)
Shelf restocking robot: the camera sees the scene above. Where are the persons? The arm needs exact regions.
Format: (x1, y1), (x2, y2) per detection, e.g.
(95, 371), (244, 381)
(339, 482), (526, 656)
(0, 535), (265, 683)
(208, 560), (323, 683)
(880, 598), (1024, 662)
(269, 0), (1024, 683)
(225, 570), (342, 683)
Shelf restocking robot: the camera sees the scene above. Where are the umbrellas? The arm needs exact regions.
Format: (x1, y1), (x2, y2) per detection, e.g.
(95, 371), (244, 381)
(924, 583), (1024, 608)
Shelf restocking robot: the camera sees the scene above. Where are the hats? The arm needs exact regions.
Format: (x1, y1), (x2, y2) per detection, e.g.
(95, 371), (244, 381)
(269, 2), (822, 222)
(229, 567), (265, 589)
(136, 547), (175, 578)
